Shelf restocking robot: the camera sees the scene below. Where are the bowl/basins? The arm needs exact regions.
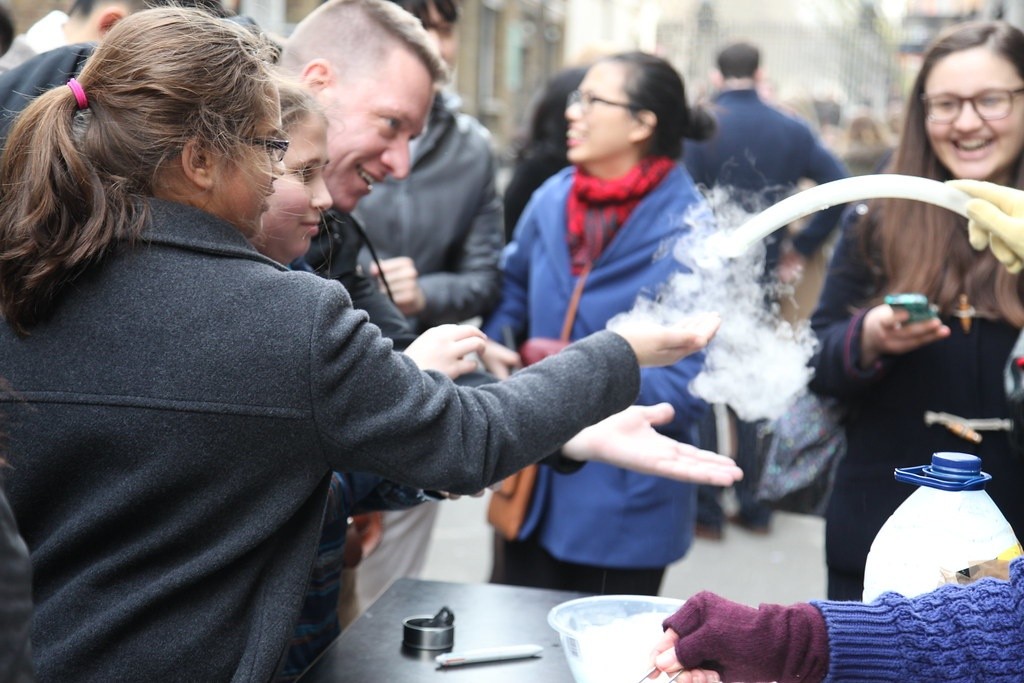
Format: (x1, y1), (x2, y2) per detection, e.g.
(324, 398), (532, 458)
(548, 595), (687, 683)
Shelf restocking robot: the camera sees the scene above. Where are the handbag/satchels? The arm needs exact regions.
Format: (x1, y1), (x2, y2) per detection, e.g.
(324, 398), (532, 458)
(483, 460), (539, 539)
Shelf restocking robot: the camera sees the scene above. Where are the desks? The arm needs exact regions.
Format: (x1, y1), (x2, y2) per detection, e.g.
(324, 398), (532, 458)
(294, 576), (599, 683)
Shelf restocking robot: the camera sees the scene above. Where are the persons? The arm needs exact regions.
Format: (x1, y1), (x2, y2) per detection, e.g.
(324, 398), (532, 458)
(0, 0), (744, 487)
(648, 557), (1024, 683)
(809, 19), (1024, 601)
(0, 0), (724, 683)
(349, 59), (505, 333)
(480, 50), (718, 597)
(0, 0), (224, 75)
(248, 82), (486, 381)
(680, 43), (852, 541)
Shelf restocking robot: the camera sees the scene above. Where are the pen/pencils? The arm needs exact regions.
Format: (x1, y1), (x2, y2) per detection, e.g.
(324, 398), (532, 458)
(435, 645), (544, 666)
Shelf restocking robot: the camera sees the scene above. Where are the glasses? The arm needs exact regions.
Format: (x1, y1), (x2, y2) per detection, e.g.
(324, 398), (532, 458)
(921, 87), (1023, 125)
(237, 134), (291, 162)
(567, 89), (636, 113)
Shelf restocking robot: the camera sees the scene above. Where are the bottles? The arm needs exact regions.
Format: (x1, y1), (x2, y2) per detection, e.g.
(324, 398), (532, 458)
(862, 453), (1023, 607)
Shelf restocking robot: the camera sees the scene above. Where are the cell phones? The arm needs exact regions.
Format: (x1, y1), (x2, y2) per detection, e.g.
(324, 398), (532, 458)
(885, 295), (939, 324)
